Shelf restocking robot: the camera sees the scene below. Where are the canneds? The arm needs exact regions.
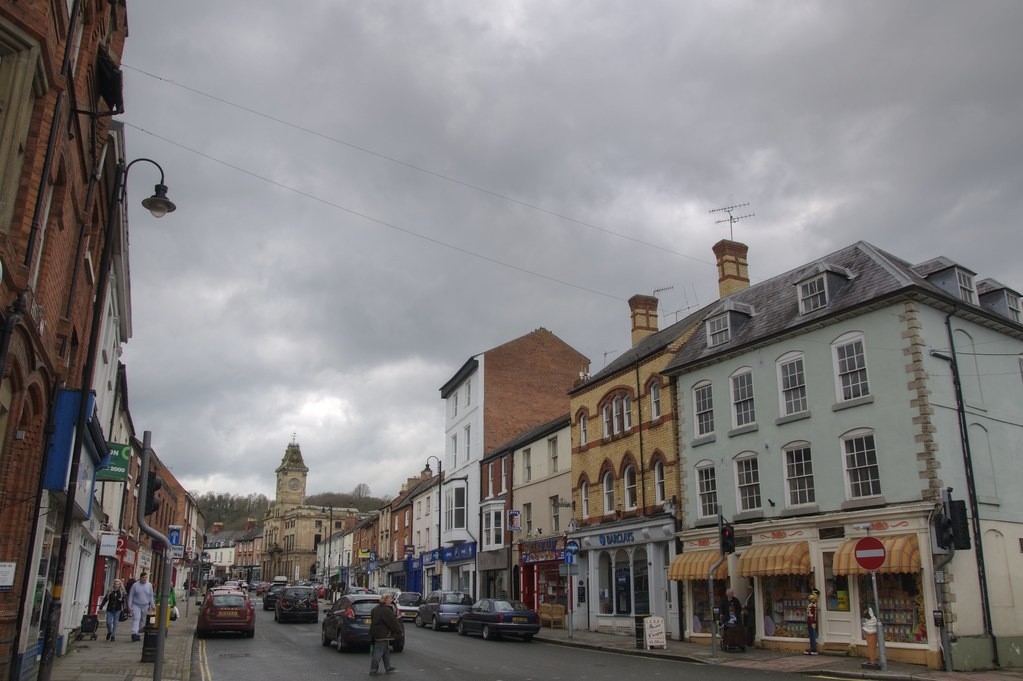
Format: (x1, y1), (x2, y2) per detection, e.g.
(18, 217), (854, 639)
(783, 600), (809, 632)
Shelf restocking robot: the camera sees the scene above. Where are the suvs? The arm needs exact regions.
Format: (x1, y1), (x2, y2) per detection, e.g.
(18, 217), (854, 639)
(273, 585), (319, 624)
(262, 576), (290, 611)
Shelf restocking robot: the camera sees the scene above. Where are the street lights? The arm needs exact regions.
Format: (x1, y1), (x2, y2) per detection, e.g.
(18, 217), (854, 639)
(318, 524), (326, 577)
(423, 456), (442, 583)
(37, 158), (177, 681)
(321, 502), (332, 583)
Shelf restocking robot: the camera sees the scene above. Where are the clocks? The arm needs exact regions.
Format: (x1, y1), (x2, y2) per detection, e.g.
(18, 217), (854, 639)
(289, 478), (300, 489)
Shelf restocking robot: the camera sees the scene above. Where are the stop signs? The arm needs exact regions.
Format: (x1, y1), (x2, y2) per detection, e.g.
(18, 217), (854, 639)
(854, 536), (886, 570)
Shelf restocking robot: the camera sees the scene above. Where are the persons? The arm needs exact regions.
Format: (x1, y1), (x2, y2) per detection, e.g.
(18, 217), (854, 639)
(368, 593), (403, 676)
(804, 588), (820, 654)
(157, 581), (176, 609)
(127, 572), (156, 641)
(182, 579), (188, 602)
(99, 579), (126, 642)
(742, 585), (756, 648)
(125, 574), (137, 617)
(495, 586), (507, 599)
(720, 588), (742, 624)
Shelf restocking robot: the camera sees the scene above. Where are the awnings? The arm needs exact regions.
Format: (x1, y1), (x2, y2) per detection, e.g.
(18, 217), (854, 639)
(832, 532), (920, 576)
(736, 541), (811, 579)
(667, 549), (728, 581)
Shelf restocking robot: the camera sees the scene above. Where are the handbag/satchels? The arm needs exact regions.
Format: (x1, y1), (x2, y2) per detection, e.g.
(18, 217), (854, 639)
(172, 606), (180, 618)
(118, 598), (128, 622)
(169, 612), (177, 621)
(370, 645), (375, 657)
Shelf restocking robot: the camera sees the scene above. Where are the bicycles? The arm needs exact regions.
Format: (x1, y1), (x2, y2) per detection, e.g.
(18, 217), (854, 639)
(280, 591), (315, 611)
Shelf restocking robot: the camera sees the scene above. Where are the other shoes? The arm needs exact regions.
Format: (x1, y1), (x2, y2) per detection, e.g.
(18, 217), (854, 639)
(106, 632), (111, 640)
(111, 636), (115, 641)
(131, 634), (141, 642)
(370, 672), (381, 676)
(387, 668), (397, 673)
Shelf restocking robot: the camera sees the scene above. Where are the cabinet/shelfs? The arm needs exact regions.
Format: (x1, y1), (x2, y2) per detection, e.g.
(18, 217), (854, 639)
(866, 605), (918, 630)
(772, 605), (816, 623)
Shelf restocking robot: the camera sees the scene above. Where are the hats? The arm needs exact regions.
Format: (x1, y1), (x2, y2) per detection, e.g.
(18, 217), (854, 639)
(727, 605), (735, 610)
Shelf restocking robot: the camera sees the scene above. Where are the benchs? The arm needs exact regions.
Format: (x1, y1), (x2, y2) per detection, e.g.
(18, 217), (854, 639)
(539, 604), (565, 628)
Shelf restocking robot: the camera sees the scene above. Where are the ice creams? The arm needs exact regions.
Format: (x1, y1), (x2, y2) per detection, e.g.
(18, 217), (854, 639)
(862, 608), (878, 666)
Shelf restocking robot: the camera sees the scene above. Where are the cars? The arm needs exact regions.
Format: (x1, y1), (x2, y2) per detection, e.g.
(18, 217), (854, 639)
(458, 598), (541, 642)
(321, 594), (405, 653)
(209, 579), (270, 597)
(394, 592), (423, 622)
(195, 588), (255, 639)
(414, 589), (473, 631)
(295, 580), (325, 599)
(340, 586), (401, 601)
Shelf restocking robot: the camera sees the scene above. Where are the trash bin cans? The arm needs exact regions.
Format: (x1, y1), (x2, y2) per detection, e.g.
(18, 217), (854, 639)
(635, 614), (652, 649)
(140, 614), (161, 663)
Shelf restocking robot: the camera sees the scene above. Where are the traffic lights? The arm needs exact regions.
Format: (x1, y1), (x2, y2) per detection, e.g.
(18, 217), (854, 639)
(935, 514), (953, 549)
(721, 525), (735, 553)
(144, 470), (162, 515)
